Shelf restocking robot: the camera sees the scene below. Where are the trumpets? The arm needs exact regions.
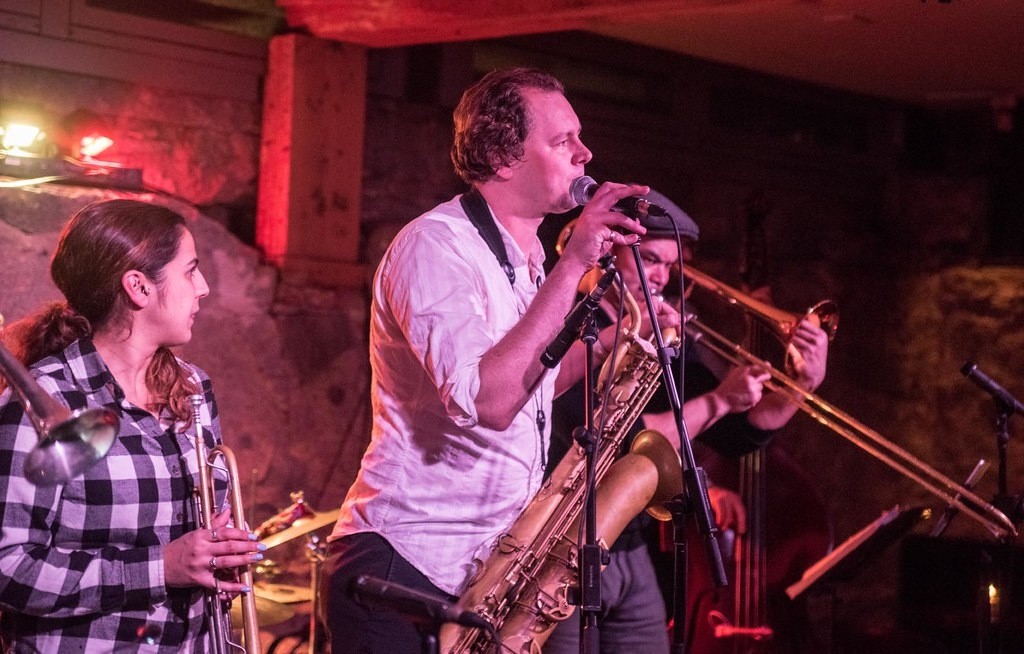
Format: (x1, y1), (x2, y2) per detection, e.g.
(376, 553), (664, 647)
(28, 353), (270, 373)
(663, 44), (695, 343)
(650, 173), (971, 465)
(0, 341), (123, 489)
(188, 390), (264, 654)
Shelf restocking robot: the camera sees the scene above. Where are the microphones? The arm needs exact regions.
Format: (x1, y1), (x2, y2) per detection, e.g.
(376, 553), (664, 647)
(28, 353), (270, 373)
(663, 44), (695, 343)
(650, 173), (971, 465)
(349, 574), (488, 627)
(932, 459), (989, 542)
(959, 361), (1024, 416)
(539, 267), (616, 369)
(569, 176), (668, 218)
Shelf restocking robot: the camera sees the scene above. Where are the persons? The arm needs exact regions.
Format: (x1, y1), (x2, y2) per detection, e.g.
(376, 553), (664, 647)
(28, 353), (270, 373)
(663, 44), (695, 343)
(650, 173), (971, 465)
(0, 198), (266, 654)
(318, 69), (685, 654)
(545, 189), (827, 654)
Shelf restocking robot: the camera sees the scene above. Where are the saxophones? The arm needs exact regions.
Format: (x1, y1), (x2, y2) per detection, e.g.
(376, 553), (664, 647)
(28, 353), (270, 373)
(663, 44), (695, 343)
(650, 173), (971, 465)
(436, 248), (689, 654)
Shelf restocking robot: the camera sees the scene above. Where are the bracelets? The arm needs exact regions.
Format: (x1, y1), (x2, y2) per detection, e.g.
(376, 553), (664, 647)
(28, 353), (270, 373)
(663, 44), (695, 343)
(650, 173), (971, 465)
(591, 339), (610, 360)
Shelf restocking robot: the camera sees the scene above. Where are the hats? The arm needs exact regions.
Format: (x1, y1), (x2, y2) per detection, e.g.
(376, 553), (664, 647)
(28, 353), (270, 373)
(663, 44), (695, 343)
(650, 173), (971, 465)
(616, 183), (701, 242)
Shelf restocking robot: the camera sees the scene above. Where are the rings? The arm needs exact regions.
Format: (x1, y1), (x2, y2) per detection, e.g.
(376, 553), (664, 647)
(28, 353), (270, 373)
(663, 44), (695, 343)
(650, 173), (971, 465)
(610, 229), (614, 241)
(209, 558), (217, 571)
(211, 529), (217, 539)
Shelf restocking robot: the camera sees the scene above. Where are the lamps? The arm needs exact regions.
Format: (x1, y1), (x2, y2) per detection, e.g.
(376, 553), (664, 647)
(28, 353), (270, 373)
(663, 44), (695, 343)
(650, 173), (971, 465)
(0, 101), (115, 163)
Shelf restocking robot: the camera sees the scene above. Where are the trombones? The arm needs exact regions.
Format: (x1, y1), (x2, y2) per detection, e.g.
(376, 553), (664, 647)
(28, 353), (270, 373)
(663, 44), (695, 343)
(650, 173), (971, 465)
(555, 205), (1020, 547)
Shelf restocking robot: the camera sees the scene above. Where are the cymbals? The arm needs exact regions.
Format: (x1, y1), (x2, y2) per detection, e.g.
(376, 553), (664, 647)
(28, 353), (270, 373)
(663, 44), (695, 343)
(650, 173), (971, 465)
(255, 509), (336, 555)
